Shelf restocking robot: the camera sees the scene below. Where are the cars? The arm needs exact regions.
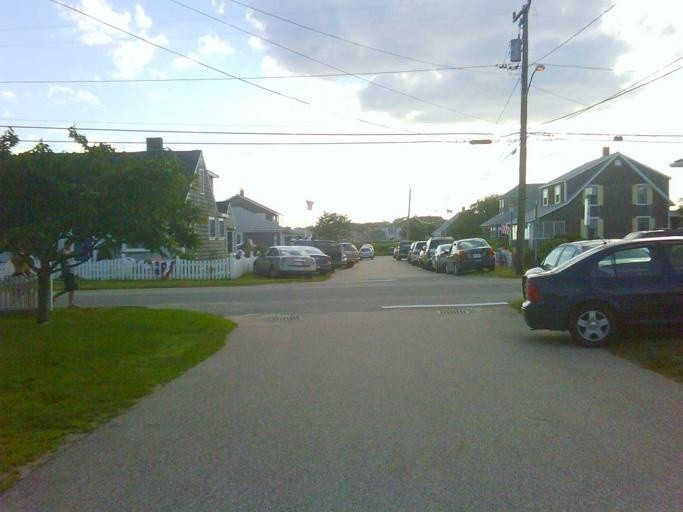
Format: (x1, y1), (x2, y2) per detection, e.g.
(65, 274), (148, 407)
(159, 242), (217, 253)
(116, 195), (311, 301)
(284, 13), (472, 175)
(519, 228), (682, 348)
(252, 237), (375, 280)
(392, 235), (497, 276)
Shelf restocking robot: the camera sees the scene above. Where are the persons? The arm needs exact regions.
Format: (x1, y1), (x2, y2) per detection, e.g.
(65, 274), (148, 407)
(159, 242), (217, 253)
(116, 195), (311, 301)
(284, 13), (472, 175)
(52, 239), (80, 309)
(10, 252), (35, 278)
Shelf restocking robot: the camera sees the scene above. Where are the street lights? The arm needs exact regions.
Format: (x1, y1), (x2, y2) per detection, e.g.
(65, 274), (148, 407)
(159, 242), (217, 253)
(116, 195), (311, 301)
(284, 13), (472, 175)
(514, 64), (546, 274)
